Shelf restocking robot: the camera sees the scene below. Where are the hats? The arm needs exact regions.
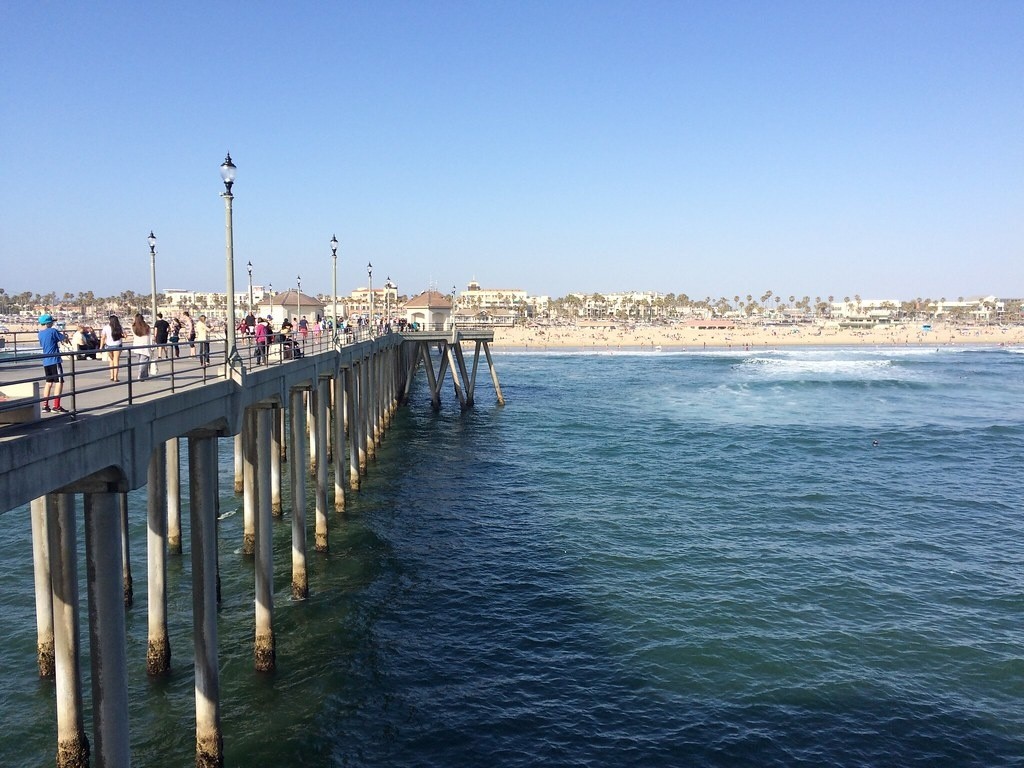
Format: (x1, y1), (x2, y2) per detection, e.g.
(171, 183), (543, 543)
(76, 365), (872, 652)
(267, 315), (273, 320)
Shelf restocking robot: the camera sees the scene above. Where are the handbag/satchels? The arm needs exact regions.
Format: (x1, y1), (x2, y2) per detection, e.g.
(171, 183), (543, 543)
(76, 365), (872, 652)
(254, 344), (261, 356)
(149, 358), (159, 375)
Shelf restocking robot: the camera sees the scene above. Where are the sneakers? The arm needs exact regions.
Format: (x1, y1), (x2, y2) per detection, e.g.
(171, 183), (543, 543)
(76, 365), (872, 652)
(51, 406), (69, 415)
(42, 407), (51, 413)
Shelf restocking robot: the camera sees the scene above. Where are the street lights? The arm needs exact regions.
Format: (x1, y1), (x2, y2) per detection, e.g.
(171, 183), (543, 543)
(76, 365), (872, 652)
(297, 275), (301, 328)
(268, 283), (272, 314)
(147, 231), (158, 337)
(219, 151), (243, 366)
(452, 285), (456, 329)
(246, 261), (253, 311)
(367, 263), (373, 327)
(330, 234), (339, 341)
(386, 276), (391, 325)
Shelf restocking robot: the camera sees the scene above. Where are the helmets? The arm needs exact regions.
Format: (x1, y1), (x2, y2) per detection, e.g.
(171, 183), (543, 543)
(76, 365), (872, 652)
(38, 314), (58, 324)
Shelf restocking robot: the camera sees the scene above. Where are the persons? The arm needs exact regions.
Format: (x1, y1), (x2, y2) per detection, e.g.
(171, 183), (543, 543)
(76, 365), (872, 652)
(154, 313), (170, 359)
(70, 325), (98, 360)
(275, 321), (303, 356)
(255, 315), (273, 365)
(236, 311), (256, 345)
(223, 318), (227, 339)
(195, 315), (214, 366)
(318, 316), (420, 335)
(282, 318), (293, 328)
(292, 317), (299, 341)
(38, 314), (69, 414)
(131, 314), (154, 382)
(312, 320), (321, 344)
(183, 312), (196, 359)
(299, 316), (309, 343)
(170, 318), (181, 358)
(99, 315), (127, 382)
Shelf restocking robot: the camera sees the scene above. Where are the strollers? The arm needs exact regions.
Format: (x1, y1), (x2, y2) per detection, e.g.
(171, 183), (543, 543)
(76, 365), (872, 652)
(278, 334), (302, 358)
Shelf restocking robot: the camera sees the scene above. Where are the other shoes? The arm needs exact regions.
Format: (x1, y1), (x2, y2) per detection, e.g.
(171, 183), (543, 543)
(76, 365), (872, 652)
(165, 356), (168, 359)
(255, 362), (265, 366)
(157, 357), (162, 359)
(86, 357), (93, 360)
(189, 357), (196, 359)
